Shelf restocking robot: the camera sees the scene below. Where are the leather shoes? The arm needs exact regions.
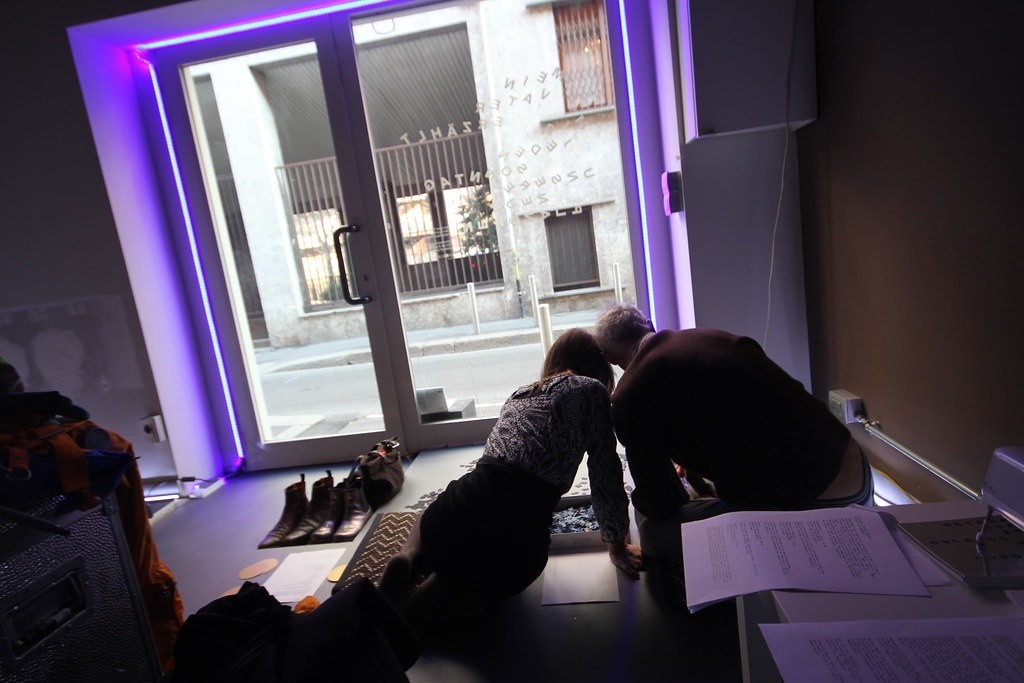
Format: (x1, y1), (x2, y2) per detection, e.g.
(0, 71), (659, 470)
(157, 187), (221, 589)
(258, 469), (373, 549)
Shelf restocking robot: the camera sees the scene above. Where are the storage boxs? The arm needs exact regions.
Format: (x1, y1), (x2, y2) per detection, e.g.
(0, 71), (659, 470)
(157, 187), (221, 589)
(0, 470), (167, 683)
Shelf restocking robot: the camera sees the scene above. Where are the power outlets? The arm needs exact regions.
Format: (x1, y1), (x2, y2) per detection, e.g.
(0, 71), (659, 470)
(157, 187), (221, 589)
(829, 387), (863, 425)
(139, 414), (167, 445)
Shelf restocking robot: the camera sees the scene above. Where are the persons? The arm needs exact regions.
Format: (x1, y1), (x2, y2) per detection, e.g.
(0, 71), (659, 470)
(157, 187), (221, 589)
(596, 306), (877, 622)
(376, 327), (646, 641)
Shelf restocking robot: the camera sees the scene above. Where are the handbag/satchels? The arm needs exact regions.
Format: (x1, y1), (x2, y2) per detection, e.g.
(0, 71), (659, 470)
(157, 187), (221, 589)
(351, 437), (406, 505)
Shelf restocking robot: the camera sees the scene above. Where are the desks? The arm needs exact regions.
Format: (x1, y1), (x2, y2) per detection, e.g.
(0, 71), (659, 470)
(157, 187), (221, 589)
(744, 500), (1024, 683)
